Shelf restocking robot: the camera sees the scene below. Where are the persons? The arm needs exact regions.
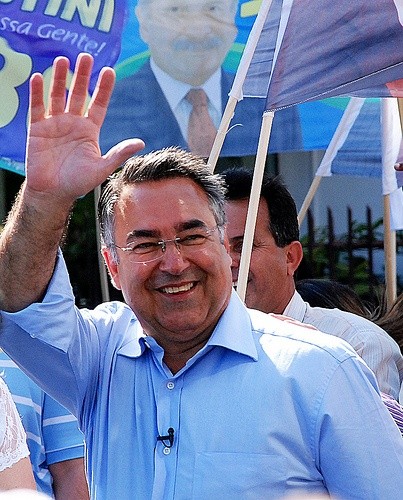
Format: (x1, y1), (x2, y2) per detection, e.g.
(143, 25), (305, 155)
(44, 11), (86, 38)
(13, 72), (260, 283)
(0, 53), (403, 500)
(294, 278), (403, 352)
(0, 375), (38, 500)
(0, 349), (90, 500)
(99, 0), (301, 156)
(211, 166), (403, 499)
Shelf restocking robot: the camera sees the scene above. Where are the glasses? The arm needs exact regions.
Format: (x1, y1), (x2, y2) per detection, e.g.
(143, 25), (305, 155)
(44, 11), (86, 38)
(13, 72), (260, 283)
(105, 225), (220, 264)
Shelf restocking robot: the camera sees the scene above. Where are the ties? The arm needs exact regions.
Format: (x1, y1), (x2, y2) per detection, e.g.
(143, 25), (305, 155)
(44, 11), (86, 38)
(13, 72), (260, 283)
(185, 88), (216, 158)
